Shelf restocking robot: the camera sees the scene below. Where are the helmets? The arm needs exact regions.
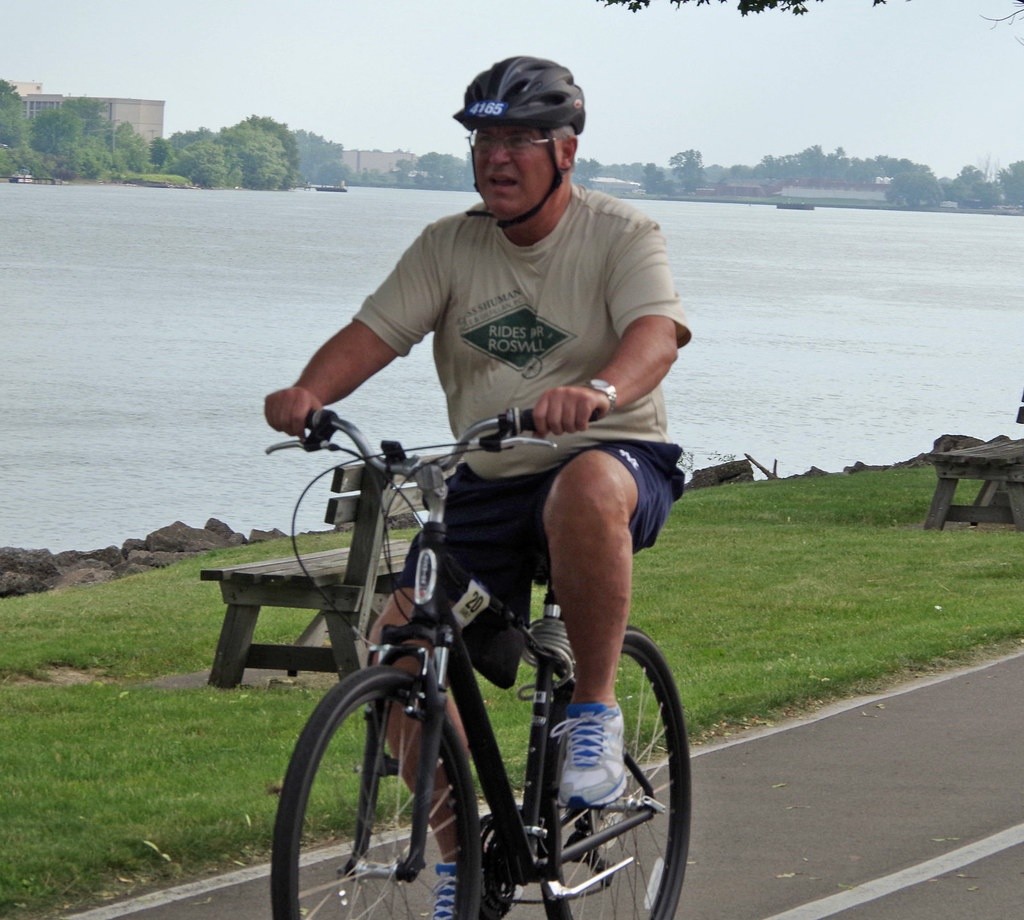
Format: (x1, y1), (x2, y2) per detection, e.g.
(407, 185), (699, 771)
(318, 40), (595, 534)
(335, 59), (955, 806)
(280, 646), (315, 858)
(453, 56), (586, 134)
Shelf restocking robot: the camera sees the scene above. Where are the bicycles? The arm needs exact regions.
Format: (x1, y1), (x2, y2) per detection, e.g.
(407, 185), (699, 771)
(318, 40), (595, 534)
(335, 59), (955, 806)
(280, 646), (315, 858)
(258, 403), (696, 919)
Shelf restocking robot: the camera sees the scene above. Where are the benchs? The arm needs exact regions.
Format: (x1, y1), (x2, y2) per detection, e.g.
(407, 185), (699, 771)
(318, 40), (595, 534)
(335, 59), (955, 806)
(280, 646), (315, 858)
(199, 453), (455, 688)
(923, 390), (1024, 533)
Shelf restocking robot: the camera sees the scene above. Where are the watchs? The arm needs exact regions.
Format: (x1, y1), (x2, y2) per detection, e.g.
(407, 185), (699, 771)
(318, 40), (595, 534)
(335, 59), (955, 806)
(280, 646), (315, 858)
(577, 374), (617, 416)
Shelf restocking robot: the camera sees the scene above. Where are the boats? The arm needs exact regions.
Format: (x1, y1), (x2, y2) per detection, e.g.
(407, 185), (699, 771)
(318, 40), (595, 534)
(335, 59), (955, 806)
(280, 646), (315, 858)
(315, 179), (349, 193)
(776, 200), (816, 212)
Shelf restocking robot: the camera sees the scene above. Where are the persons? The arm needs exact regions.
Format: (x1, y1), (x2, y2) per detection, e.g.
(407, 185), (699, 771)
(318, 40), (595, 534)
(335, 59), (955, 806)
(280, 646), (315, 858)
(259, 54), (693, 920)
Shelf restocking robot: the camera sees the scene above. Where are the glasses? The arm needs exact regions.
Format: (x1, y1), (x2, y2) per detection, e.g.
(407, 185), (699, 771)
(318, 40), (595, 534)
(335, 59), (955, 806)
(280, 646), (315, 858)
(467, 133), (557, 154)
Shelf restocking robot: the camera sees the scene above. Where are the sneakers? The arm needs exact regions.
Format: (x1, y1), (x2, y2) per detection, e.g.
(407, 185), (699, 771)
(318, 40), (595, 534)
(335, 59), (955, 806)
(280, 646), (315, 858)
(432, 862), (458, 920)
(549, 703), (627, 807)
